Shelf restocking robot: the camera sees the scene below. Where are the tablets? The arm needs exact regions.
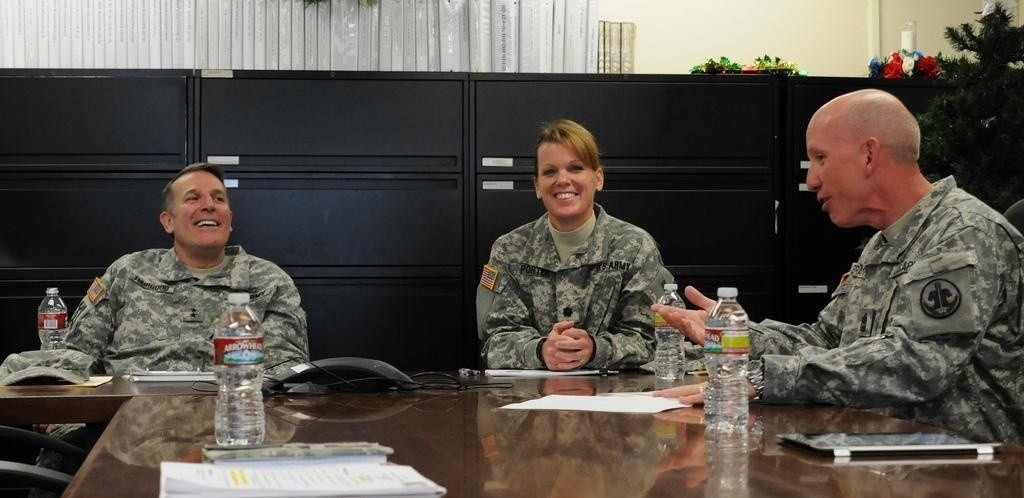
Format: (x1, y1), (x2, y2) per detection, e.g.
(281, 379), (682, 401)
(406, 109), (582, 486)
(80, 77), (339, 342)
(775, 432), (1004, 458)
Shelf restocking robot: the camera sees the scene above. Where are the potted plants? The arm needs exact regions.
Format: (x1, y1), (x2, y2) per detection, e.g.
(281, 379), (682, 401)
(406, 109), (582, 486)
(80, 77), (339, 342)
(687, 54), (809, 76)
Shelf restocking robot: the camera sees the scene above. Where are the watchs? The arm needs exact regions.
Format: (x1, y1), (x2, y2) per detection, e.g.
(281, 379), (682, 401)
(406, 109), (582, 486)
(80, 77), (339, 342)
(744, 359), (766, 398)
(749, 410), (764, 442)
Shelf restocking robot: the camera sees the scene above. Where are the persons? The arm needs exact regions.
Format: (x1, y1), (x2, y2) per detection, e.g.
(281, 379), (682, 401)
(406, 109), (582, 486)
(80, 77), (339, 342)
(475, 117), (676, 373)
(652, 407), (764, 488)
(476, 378), (687, 496)
(27, 164), (311, 497)
(104, 396), (301, 470)
(650, 88), (1024, 448)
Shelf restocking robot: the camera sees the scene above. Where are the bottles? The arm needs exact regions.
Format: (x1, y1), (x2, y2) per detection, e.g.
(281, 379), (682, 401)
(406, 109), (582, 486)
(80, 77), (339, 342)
(901, 19), (918, 51)
(702, 287), (751, 428)
(214, 292), (266, 447)
(37, 287), (69, 351)
(704, 428), (749, 498)
(654, 283), (687, 381)
(654, 381), (686, 460)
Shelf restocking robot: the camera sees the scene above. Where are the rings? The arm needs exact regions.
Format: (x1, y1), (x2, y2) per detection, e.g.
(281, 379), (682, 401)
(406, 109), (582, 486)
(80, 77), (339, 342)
(578, 353), (584, 363)
(697, 384), (703, 392)
(697, 418), (704, 425)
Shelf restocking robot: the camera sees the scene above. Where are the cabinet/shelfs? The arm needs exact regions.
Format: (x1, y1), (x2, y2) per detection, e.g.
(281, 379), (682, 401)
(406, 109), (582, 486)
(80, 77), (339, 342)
(3, 72), (190, 164)
(2, 177), (176, 263)
(793, 276), (840, 325)
(302, 286), (467, 355)
(206, 179), (463, 269)
(474, 81), (784, 163)
(2, 282), (82, 351)
(789, 83), (1017, 174)
(476, 179), (783, 271)
(792, 185), (1016, 266)
(472, 282), (783, 367)
(203, 77), (463, 169)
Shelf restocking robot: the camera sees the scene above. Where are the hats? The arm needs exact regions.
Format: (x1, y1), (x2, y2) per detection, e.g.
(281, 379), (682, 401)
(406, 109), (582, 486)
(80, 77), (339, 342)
(1, 348), (96, 384)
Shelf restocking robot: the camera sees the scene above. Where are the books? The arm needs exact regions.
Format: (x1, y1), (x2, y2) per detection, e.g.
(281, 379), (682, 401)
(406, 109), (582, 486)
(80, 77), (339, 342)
(1, 0), (633, 74)
(125, 370), (216, 383)
(483, 367), (621, 378)
(157, 436), (449, 497)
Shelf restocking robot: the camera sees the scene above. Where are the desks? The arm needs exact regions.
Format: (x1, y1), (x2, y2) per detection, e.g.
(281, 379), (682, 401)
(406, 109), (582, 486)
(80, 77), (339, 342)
(0, 372), (1024, 498)
